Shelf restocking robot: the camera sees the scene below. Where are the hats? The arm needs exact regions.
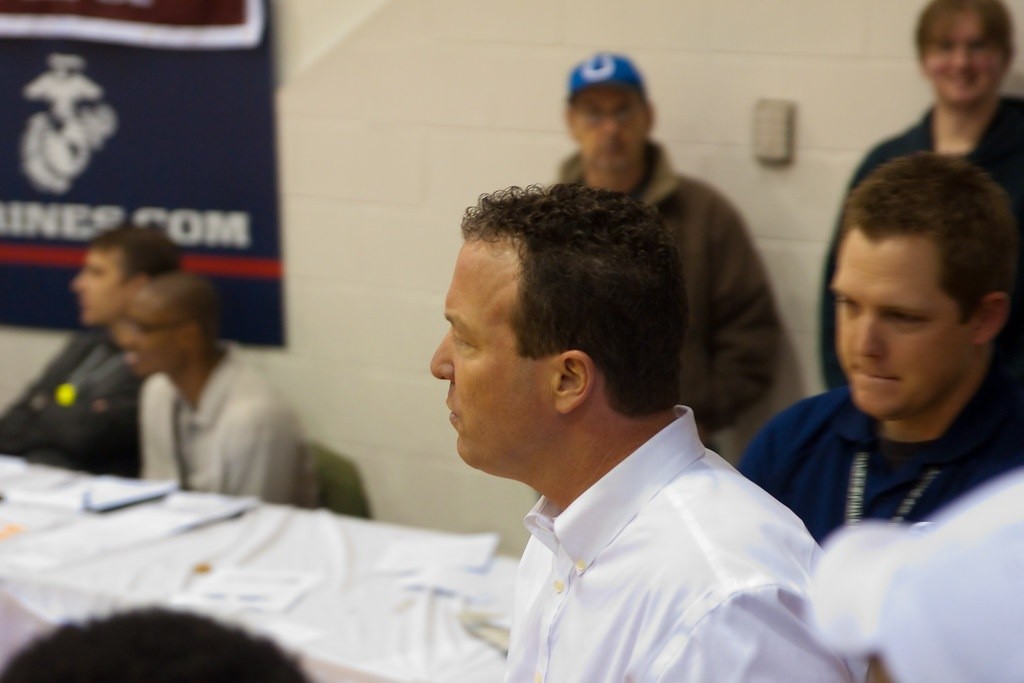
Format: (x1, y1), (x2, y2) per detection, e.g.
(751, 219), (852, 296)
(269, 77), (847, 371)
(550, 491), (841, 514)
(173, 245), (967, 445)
(568, 53), (646, 105)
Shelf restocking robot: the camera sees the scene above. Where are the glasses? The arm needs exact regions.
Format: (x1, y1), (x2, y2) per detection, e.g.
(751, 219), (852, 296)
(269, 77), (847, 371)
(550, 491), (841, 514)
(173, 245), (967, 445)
(576, 102), (647, 126)
(121, 311), (201, 336)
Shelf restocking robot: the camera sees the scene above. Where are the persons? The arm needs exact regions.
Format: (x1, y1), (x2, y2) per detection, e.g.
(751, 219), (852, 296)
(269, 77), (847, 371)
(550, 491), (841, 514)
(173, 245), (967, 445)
(430, 183), (896, 683)
(735, 155), (1024, 683)
(120, 272), (322, 513)
(553, 51), (783, 462)
(0, 227), (182, 481)
(813, 0), (1023, 397)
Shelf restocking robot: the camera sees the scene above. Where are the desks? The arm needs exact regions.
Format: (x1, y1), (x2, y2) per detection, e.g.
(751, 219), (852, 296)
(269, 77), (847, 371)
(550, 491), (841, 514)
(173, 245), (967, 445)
(0, 456), (514, 682)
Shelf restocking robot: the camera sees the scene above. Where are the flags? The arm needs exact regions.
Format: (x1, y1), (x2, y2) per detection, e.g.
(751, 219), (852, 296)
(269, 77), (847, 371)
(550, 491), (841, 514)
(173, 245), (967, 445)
(0, 3), (293, 353)
